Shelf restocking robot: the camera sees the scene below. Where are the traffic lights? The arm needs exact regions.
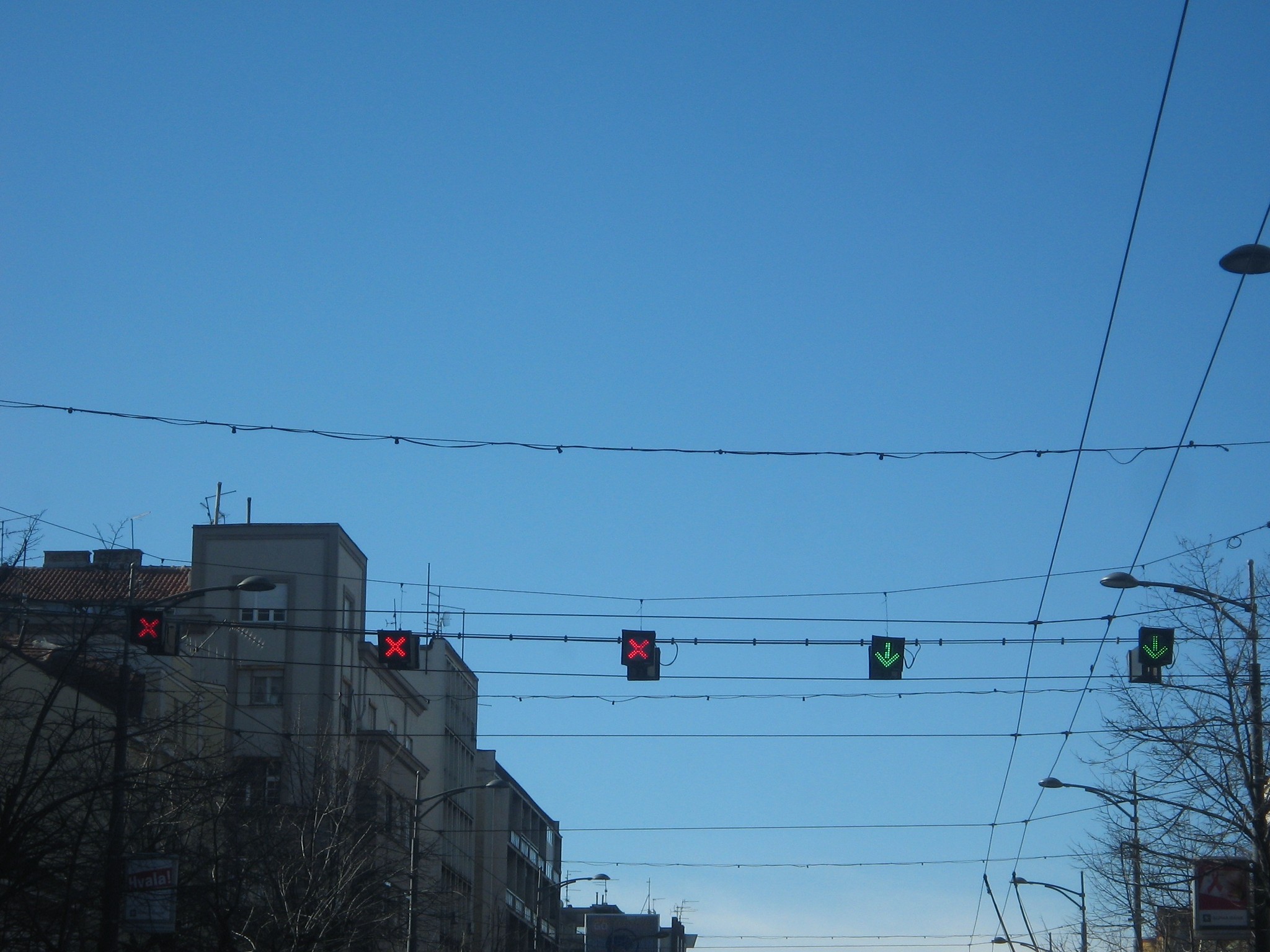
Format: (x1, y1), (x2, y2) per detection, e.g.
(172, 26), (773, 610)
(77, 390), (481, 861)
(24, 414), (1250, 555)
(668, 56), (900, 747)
(377, 629), (414, 663)
(867, 632), (906, 676)
(1135, 620), (1177, 669)
(618, 628), (661, 665)
(129, 608), (169, 648)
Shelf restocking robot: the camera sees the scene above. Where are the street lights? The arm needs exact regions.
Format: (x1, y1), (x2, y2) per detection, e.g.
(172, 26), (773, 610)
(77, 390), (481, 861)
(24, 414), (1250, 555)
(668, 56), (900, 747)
(1038, 768), (1145, 951)
(1099, 558), (1269, 952)
(992, 930), (1053, 951)
(101, 575), (278, 949)
(405, 769), (511, 952)
(534, 863), (611, 952)
(1009, 870), (1089, 952)
